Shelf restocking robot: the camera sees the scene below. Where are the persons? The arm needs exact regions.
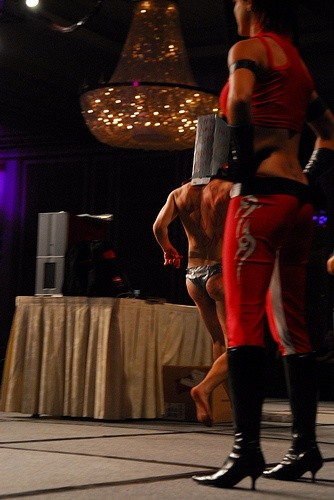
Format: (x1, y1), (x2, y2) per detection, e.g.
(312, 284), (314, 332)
(193, 0), (334, 491)
(153, 163), (237, 426)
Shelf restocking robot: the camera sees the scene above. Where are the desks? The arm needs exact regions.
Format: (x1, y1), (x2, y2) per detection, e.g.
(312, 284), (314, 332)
(0, 295), (214, 422)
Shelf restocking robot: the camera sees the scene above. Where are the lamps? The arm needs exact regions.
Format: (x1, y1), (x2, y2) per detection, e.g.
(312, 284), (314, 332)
(77, 0), (221, 152)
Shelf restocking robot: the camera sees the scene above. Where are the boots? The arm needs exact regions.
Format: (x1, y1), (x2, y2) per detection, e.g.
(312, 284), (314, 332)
(191, 345), (269, 490)
(262, 351), (324, 483)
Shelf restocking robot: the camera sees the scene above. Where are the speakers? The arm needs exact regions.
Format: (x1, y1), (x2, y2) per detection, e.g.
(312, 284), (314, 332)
(36, 212), (117, 297)
